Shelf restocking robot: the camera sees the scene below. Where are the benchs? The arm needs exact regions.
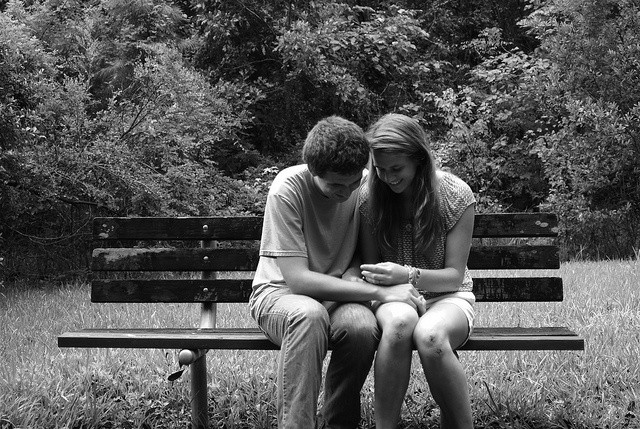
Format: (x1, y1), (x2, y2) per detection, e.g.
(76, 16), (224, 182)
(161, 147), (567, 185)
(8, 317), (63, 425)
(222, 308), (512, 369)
(57, 213), (585, 429)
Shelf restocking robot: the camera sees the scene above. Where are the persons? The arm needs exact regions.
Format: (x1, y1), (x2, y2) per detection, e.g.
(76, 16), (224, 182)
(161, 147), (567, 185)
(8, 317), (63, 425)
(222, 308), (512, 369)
(248, 115), (426, 428)
(358, 112), (475, 428)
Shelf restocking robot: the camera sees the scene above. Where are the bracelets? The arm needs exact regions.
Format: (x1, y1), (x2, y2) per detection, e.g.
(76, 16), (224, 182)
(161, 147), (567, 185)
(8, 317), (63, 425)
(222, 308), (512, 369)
(405, 263), (413, 282)
(413, 266), (420, 285)
